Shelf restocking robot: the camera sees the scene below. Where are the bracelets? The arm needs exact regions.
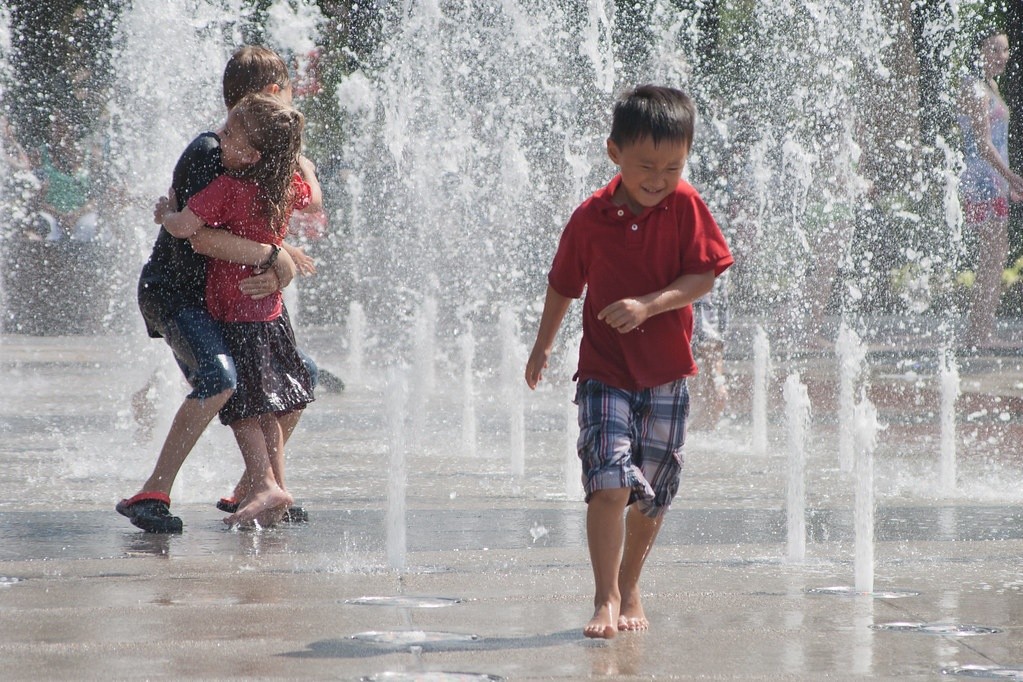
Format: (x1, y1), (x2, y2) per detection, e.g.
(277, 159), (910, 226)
(260, 243), (281, 269)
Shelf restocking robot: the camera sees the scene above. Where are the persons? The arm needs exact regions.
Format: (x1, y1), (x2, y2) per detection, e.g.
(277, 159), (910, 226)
(153, 92), (323, 526)
(688, 272), (729, 431)
(1, 109), (96, 334)
(117, 42), (318, 534)
(953, 25), (1023, 344)
(526, 87), (737, 634)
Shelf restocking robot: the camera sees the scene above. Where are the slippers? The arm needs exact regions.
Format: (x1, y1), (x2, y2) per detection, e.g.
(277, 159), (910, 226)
(217, 497), (308, 524)
(116, 492), (183, 534)
